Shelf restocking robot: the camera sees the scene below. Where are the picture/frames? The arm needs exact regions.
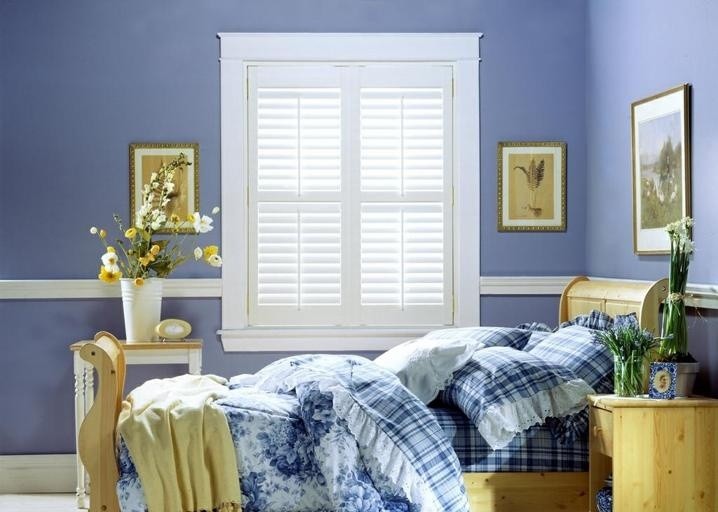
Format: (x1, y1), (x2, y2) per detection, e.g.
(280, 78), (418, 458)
(629, 82), (693, 257)
(496, 140), (568, 232)
(128, 142), (199, 235)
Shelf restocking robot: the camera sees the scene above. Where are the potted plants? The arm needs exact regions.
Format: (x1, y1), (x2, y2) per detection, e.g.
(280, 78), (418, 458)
(589, 323), (674, 399)
(645, 216), (701, 401)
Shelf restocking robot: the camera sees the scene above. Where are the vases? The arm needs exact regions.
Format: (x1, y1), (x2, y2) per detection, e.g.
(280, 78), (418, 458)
(118, 277), (164, 345)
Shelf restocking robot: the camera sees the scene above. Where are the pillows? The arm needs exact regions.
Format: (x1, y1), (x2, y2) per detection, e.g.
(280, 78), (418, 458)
(530, 308), (641, 440)
(445, 347), (598, 450)
(516, 321), (555, 350)
(423, 325), (533, 353)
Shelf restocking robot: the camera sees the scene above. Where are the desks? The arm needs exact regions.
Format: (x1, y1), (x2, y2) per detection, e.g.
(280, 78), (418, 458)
(67, 338), (204, 512)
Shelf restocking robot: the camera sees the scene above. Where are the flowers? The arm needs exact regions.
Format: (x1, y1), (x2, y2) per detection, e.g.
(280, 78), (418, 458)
(86, 151), (224, 289)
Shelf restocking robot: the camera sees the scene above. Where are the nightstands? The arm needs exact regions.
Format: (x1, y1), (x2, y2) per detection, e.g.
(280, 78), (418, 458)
(586, 391), (718, 511)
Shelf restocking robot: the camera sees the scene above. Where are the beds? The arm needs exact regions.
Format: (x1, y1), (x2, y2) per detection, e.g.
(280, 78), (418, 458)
(78, 276), (674, 512)
